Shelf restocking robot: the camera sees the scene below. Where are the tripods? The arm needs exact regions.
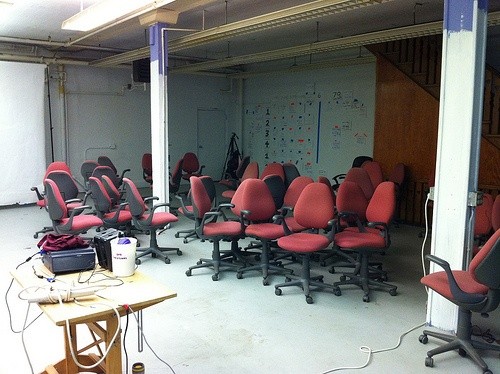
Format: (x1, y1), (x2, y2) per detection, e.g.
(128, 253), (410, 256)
(221, 132), (243, 180)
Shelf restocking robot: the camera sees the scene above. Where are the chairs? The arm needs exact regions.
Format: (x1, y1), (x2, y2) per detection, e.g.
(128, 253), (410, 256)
(418, 190), (500, 374)
(30, 152), (406, 305)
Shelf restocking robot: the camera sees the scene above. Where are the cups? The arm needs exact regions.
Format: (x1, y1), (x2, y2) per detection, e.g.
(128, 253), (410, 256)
(110, 237), (137, 276)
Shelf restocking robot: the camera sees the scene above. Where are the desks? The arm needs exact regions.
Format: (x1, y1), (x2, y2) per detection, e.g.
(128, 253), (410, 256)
(8, 266), (177, 374)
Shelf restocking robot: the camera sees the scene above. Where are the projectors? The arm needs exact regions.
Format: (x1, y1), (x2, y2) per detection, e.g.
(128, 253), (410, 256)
(41, 245), (95, 273)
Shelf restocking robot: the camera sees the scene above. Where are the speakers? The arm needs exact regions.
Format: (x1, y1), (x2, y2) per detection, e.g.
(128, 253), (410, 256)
(133, 58), (150, 83)
